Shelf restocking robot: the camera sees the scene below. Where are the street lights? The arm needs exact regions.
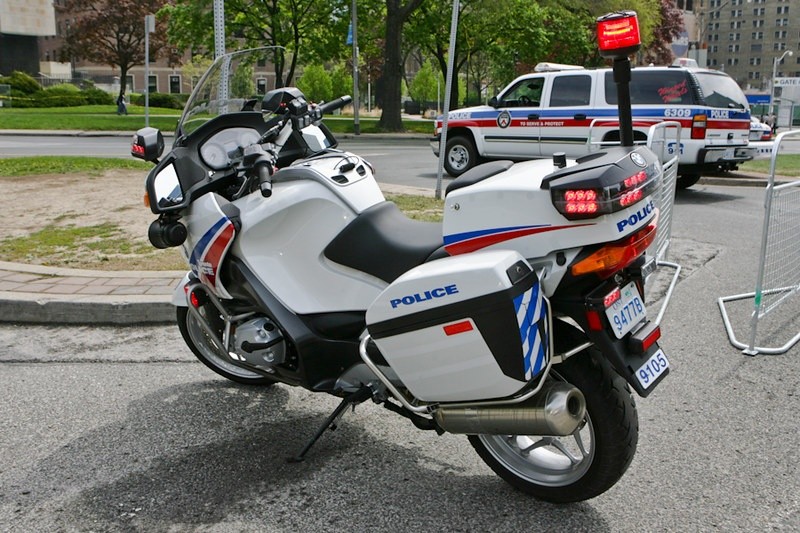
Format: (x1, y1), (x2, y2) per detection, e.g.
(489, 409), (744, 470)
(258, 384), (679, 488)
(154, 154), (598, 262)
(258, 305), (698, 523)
(768, 49), (794, 132)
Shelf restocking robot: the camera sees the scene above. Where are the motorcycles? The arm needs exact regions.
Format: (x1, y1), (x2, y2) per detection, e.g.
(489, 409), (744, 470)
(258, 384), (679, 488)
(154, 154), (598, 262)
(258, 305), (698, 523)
(130, 8), (675, 503)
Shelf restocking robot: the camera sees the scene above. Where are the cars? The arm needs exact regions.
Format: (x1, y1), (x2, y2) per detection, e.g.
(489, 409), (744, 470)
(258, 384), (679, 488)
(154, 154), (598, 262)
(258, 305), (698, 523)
(749, 115), (774, 142)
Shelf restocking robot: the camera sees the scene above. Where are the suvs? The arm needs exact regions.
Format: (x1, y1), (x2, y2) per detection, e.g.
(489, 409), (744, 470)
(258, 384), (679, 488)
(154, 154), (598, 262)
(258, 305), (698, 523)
(429, 57), (754, 196)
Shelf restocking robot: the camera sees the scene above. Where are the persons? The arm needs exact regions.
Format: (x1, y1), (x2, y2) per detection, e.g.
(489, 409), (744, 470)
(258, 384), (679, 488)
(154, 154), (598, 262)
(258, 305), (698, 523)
(760, 113), (777, 134)
(118, 93), (128, 116)
